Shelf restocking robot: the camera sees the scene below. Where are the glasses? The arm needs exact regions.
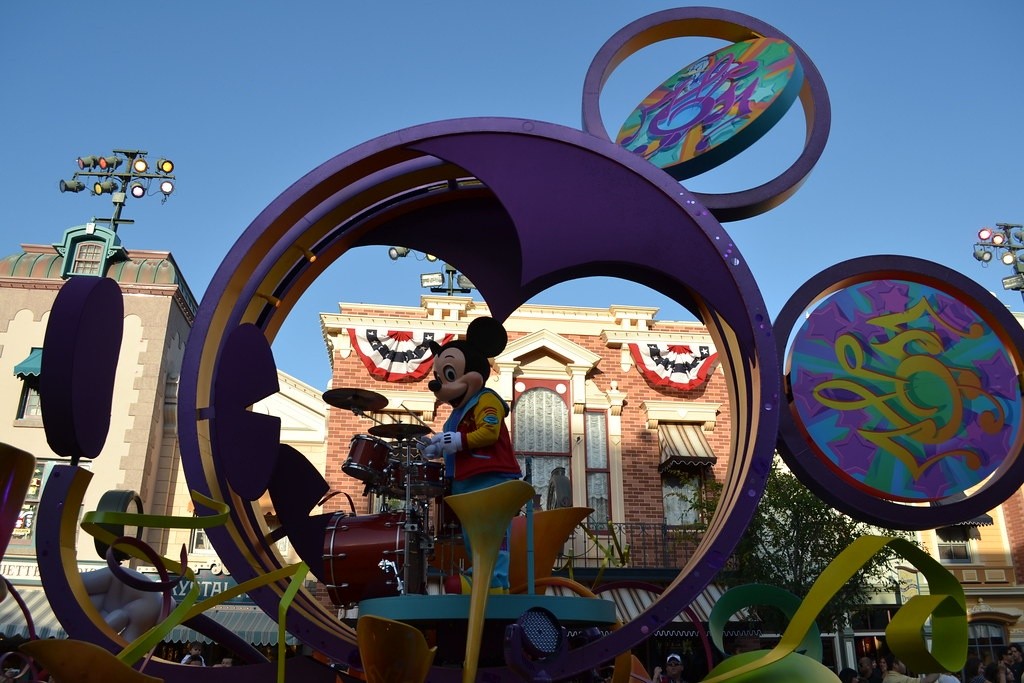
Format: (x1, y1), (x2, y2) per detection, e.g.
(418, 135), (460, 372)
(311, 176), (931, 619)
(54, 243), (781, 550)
(667, 663), (682, 666)
(1005, 653), (1012, 656)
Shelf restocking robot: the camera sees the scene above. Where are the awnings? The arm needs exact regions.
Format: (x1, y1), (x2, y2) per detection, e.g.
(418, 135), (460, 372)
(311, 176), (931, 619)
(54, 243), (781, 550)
(658, 423), (717, 474)
(14, 348), (43, 381)
(952, 514), (994, 527)
(338, 576), (764, 638)
(0, 586), (300, 647)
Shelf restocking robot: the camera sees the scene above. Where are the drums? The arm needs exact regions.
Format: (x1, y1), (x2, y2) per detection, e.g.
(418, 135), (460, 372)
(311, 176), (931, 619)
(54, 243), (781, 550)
(385, 461), (446, 498)
(322, 511), (423, 604)
(341, 434), (393, 482)
(366, 457), (408, 498)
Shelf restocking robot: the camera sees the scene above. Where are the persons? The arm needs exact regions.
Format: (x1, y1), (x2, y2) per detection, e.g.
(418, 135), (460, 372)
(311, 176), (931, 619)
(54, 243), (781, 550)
(838, 643), (1024, 683)
(652, 654), (689, 683)
(181, 641), (232, 667)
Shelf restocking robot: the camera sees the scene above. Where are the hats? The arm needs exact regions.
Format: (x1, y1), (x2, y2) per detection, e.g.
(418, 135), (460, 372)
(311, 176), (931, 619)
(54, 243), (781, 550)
(666, 654), (682, 663)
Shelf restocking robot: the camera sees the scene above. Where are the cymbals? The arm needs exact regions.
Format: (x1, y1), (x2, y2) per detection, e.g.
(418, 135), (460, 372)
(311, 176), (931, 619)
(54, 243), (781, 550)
(322, 387), (388, 413)
(368, 424), (430, 440)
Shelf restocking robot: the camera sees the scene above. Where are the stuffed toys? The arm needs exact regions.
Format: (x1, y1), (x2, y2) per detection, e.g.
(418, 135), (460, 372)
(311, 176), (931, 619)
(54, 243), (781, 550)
(417, 317), (523, 594)
